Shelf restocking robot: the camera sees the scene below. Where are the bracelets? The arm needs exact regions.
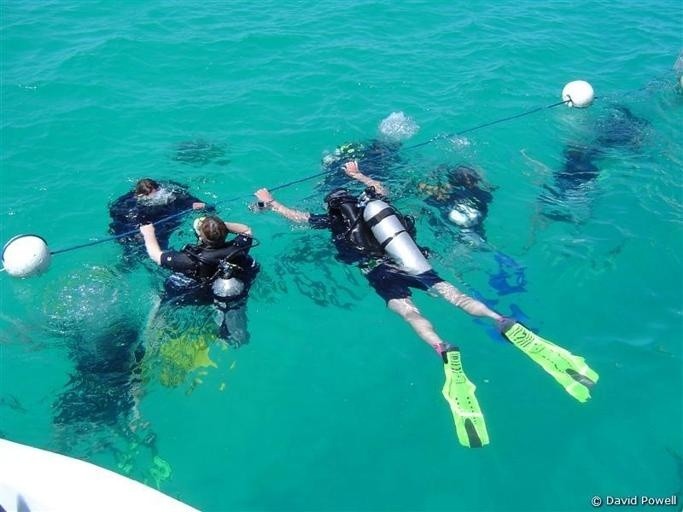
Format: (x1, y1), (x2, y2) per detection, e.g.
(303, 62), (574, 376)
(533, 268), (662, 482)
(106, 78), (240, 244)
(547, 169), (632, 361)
(265, 198), (274, 207)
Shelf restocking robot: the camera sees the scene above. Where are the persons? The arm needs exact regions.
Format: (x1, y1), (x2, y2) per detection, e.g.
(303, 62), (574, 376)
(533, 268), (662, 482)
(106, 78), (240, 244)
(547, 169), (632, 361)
(99, 178), (216, 276)
(138, 215), (254, 345)
(252, 161), (600, 451)
(415, 167), (493, 255)
